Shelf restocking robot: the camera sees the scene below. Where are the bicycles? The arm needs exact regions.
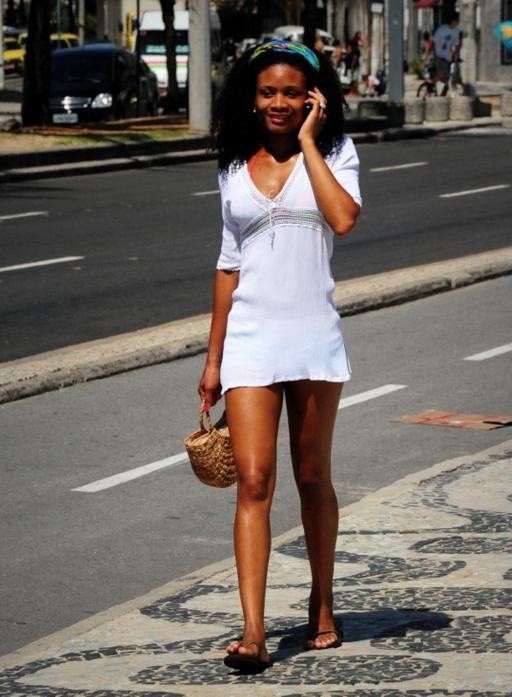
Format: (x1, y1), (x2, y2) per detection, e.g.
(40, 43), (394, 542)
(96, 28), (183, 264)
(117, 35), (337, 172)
(416, 60), (463, 97)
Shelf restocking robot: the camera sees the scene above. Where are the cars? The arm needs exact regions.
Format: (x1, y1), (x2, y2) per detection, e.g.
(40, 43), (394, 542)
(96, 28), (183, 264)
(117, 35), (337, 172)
(2, 34), (77, 67)
(39, 45), (160, 122)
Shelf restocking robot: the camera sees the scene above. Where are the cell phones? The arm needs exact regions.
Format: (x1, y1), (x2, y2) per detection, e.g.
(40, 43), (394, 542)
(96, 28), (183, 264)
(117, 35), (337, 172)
(303, 96), (314, 119)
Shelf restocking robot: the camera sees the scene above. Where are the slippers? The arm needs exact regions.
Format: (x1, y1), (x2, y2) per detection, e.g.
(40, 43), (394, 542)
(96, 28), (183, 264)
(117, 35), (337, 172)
(225, 643), (272, 671)
(305, 630), (343, 650)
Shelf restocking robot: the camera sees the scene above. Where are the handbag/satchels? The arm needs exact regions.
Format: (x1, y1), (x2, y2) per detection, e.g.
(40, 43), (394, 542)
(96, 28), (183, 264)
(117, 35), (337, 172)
(185, 400), (237, 488)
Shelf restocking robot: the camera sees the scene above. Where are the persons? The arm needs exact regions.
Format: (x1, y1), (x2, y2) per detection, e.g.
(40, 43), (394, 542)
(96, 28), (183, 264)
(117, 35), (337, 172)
(316, 12), (461, 98)
(198, 39), (364, 672)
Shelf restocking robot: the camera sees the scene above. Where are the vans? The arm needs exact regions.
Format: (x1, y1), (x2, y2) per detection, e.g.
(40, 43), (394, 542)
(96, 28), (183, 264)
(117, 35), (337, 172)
(277, 26), (342, 55)
(135, 10), (222, 91)
